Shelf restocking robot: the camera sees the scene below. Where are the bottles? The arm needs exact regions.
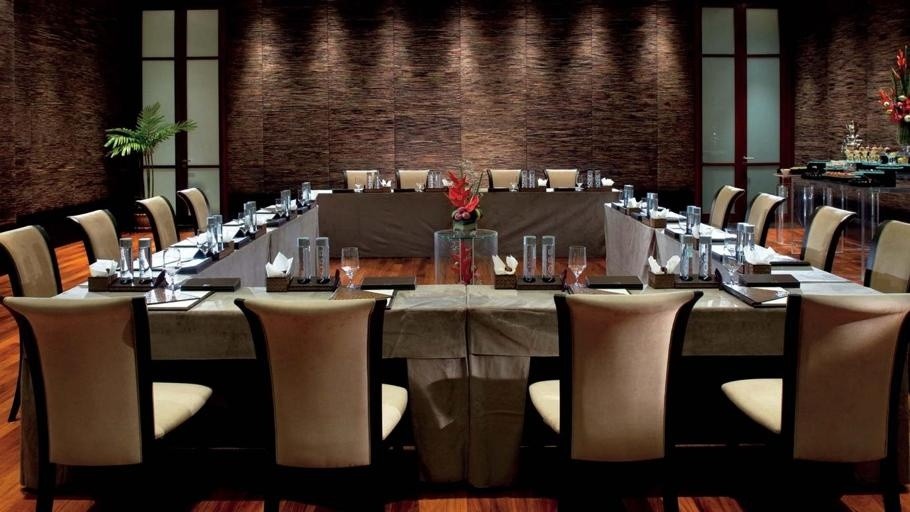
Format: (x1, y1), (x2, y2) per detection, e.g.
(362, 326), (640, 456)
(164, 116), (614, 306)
(687, 205), (694, 232)
(522, 170), (528, 189)
(699, 236), (712, 280)
(138, 238), (152, 282)
(541, 236), (555, 283)
(435, 172), (440, 188)
(207, 216), (219, 256)
(246, 202), (257, 234)
(281, 191), (287, 214)
(298, 237), (311, 282)
(523, 235), (536, 283)
(588, 170), (593, 188)
(646, 192), (651, 219)
(307, 182), (311, 201)
(529, 170), (535, 189)
(624, 184), (634, 208)
(374, 172), (379, 190)
(649, 193), (658, 213)
(681, 235), (692, 280)
(736, 222), (748, 266)
(213, 215), (224, 253)
(286, 189), (291, 210)
(428, 172), (434, 190)
(303, 184), (307, 204)
(367, 173), (373, 190)
(691, 208), (700, 238)
(744, 225), (754, 251)
(595, 170), (600, 189)
(316, 236), (330, 284)
(244, 204), (250, 234)
(120, 238), (133, 284)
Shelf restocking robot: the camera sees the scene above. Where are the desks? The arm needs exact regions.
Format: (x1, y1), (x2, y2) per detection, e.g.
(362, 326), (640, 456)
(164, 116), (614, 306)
(51, 283), (467, 495)
(116, 201), (320, 285)
(604, 201), (857, 284)
(309, 188), (622, 257)
(467, 271), (883, 494)
(775, 168), (910, 282)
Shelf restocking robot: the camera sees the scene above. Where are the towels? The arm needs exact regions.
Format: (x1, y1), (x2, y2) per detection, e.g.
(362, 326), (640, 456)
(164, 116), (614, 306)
(442, 178), (454, 186)
(601, 177), (615, 186)
(537, 177), (548, 186)
(742, 246), (777, 266)
(492, 255), (519, 275)
(265, 252), (293, 277)
(88, 258), (118, 278)
(649, 208), (669, 219)
(692, 223), (712, 239)
(647, 256), (681, 275)
(380, 179), (392, 187)
(626, 197), (643, 209)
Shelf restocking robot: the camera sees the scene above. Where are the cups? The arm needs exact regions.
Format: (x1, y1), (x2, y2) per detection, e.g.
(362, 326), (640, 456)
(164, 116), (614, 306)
(339, 247), (360, 291)
(620, 189), (627, 208)
(275, 198), (286, 218)
(678, 210), (689, 237)
(508, 177), (518, 192)
(162, 246), (182, 291)
(297, 190), (306, 207)
(575, 174), (584, 191)
(416, 183), (425, 191)
(567, 245), (587, 292)
(353, 174), (362, 193)
(196, 228), (209, 259)
(642, 197), (649, 212)
(238, 212), (249, 236)
(721, 242), (746, 288)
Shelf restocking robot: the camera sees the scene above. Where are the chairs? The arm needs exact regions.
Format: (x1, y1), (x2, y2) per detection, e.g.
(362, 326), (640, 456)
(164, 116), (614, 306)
(0, 296), (214, 512)
(137, 193), (180, 253)
(234, 295), (411, 512)
(529, 290), (706, 512)
(486, 168), (522, 191)
(744, 193), (785, 245)
(396, 170), (430, 189)
(344, 170), (380, 188)
(864, 220), (910, 293)
(68, 210), (123, 265)
(543, 168), (580, 190)
(708, 185), (745, 229)
(800, 206), (857, 271)
(0, 223), (62, 496)
(177, 186), (212, 235)
(723, 294), (910, 512)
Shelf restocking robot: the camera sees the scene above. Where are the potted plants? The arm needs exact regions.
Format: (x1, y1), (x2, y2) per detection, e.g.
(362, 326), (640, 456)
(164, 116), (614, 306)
(104, 103), (199, 229)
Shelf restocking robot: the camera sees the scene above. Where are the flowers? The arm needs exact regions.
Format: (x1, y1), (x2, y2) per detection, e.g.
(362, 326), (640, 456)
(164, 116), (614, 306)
(879, 44), (910, 147)
(438, 171), (491, 230)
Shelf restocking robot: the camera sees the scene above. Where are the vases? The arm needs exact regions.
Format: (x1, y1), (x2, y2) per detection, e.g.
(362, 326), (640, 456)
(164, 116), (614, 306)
(434, 228), (498, 286)
(898, 119), (910, 156)
